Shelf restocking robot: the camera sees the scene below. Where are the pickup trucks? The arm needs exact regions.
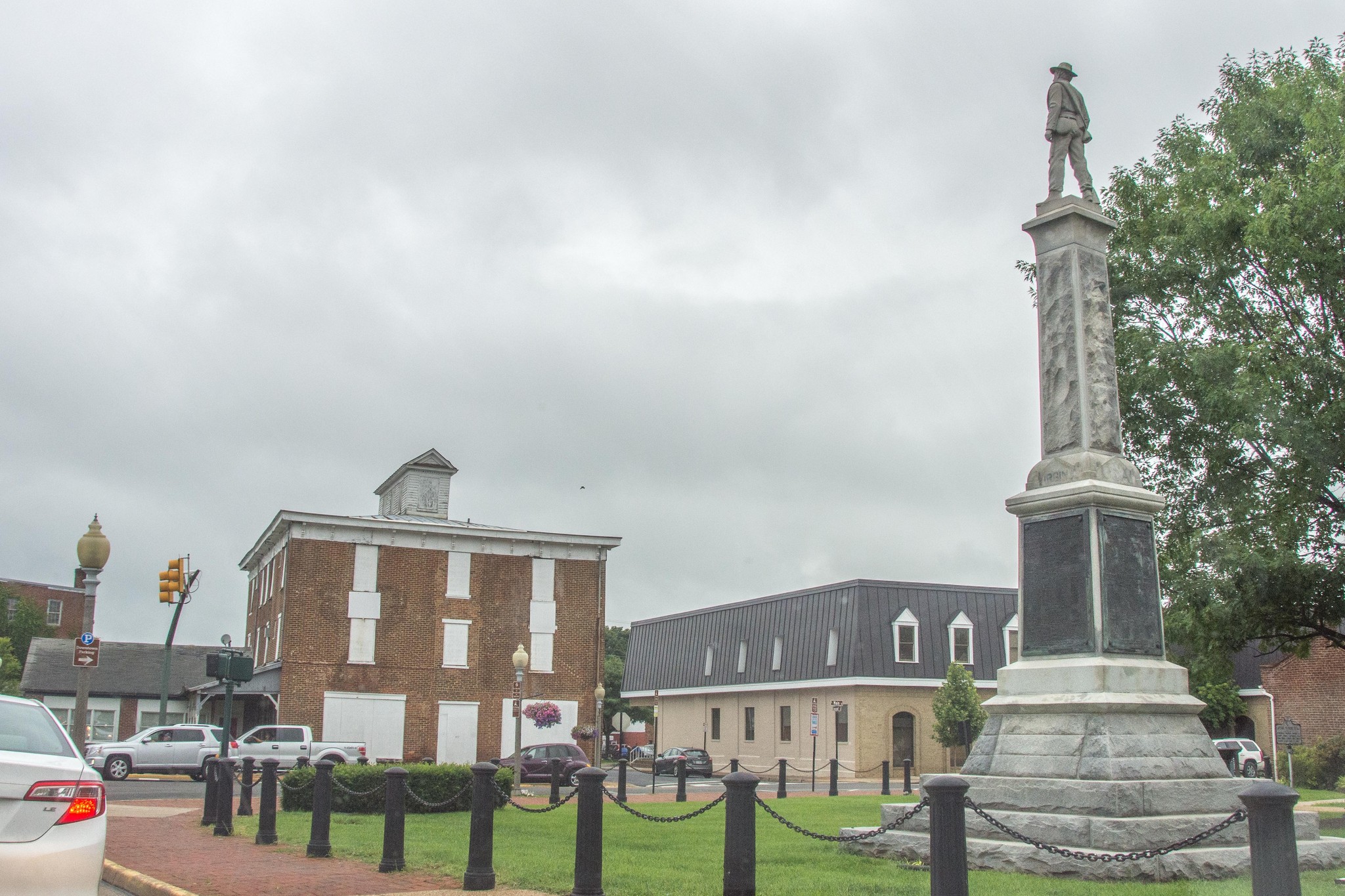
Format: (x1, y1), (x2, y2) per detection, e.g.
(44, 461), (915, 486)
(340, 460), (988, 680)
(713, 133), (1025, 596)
(216, 725), (367, 773)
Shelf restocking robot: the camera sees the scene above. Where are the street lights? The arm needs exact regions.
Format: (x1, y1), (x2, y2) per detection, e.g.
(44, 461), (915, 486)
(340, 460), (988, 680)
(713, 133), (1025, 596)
(593, 682), (605, 769)
(70, 513), (110, 756)
(510, 640), (529, 795)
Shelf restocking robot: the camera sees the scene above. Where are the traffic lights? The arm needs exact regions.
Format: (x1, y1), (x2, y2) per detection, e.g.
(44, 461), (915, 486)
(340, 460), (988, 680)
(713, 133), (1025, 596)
(159, 571), (175, 605)
(168, 558), (186, 592)
(206, 652), (229, 678)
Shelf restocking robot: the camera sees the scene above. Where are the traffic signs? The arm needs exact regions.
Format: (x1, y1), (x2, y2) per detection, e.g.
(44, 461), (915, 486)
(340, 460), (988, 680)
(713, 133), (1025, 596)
(73, 638), (101, 667)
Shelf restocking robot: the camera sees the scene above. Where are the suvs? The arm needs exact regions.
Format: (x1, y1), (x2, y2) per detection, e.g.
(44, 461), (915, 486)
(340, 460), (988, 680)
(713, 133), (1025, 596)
(85, 723), (239, 782)
(1211, 738), (1266, 780)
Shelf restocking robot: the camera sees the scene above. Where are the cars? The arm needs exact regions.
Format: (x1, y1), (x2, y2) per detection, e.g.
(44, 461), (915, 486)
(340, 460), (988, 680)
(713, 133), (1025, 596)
(0, 694), (109, 896)
(476, 743), (591, 788)
(628, 744), (654, 762)
(61, 722), (114, 742)
(652, 747), (713, 779)
(600, 744), (632, 759)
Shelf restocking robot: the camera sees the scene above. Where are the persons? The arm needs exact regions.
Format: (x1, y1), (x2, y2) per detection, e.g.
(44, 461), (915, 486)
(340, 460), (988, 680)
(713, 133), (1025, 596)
(253, 731), (270, 743)
(150, 733), (171, 741)
(1036, 61), (1093, 206)
(615, 742), (638, 757)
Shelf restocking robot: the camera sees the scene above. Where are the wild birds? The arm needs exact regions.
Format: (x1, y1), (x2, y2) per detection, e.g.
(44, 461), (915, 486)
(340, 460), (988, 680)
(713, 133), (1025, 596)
(580, 486), (585, 490)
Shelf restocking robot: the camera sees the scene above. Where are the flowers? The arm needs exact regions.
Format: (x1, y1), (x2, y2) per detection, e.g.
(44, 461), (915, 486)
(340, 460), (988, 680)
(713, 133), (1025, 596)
(522, 701), (562, 731)
(570, 724), (598, 741)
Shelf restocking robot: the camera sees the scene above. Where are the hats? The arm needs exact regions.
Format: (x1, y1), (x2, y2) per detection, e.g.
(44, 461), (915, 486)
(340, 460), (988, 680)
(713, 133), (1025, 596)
(165, 732), (172, 738)
